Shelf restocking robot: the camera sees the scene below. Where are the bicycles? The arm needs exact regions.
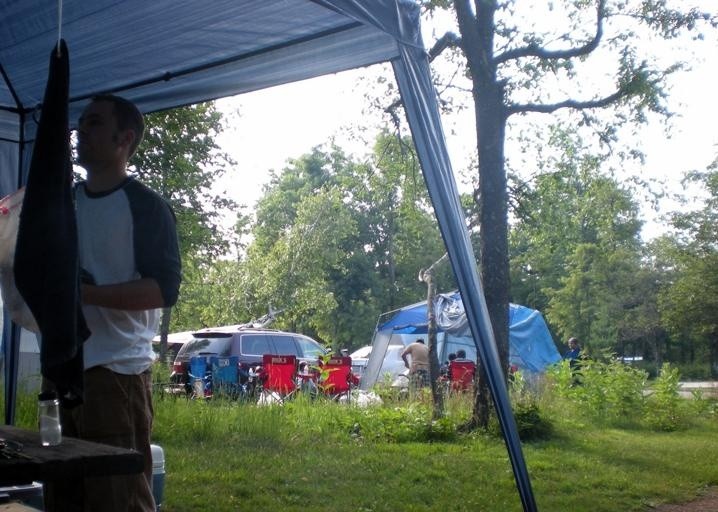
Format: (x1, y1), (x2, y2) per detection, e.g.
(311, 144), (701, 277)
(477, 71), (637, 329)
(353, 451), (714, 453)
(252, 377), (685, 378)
(187, 373), (212, 404)
(157, 383), (188, 402)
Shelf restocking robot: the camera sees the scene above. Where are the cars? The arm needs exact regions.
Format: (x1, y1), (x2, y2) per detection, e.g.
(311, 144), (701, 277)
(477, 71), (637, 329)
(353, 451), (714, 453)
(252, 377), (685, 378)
(170, 323), (372, 396)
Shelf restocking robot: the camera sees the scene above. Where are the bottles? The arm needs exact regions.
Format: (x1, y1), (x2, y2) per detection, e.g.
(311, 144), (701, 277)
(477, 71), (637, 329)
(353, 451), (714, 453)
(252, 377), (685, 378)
(38, 390), (62, 446)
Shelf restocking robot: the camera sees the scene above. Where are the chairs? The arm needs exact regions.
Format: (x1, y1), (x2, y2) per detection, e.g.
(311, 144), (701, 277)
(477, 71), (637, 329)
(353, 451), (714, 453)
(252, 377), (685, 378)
(155, 356), (240, 402)
(263, 354), (358, 404)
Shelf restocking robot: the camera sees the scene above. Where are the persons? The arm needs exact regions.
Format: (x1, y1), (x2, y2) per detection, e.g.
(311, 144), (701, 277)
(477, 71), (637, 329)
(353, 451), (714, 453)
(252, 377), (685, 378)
(563, 337), (585, 388)
(402, 338), (476, 397)
(46, 94), (183, 512)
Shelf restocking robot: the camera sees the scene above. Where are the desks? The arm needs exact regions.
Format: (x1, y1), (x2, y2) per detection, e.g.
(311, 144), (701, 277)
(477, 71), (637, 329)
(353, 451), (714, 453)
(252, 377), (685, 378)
(0, 422), (143, 512)
